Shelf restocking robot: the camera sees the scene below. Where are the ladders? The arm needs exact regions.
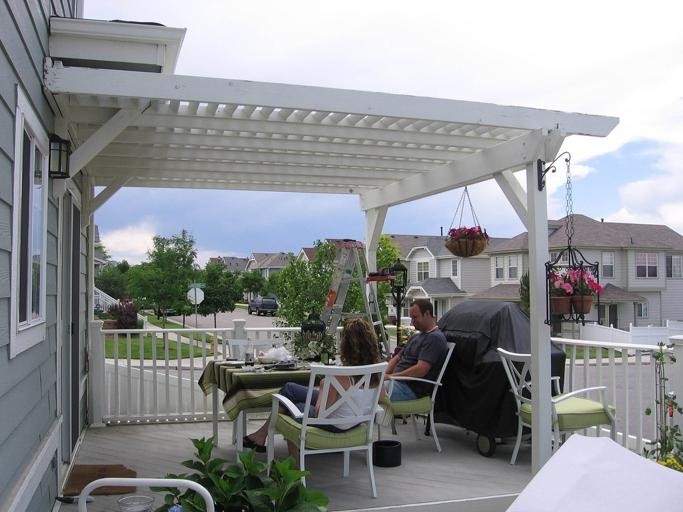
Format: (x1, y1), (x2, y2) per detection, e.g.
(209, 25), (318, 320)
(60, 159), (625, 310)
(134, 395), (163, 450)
(320, 239), (392, 363)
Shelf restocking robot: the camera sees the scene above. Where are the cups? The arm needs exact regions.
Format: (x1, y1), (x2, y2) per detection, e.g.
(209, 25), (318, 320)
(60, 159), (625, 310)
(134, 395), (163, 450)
(117, 495), (154, 512)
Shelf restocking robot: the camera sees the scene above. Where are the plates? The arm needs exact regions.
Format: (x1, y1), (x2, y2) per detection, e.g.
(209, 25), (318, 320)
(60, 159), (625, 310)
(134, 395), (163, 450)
(222, 360), (244, 368)
(271, 362), (302, 371)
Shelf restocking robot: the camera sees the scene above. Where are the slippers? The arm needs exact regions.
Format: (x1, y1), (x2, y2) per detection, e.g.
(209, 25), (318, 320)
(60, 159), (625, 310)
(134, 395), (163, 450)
(234, 436), (266, 452)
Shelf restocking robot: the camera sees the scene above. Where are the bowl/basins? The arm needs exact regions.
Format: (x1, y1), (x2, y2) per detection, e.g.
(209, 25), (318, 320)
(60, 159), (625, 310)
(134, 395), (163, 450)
(257, 356), (275, 365)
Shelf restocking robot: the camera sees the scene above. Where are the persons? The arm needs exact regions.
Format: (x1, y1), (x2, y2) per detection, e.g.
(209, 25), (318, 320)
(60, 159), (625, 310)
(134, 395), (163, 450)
(384, 299), (447, 401)
(233, 318), (385, 472)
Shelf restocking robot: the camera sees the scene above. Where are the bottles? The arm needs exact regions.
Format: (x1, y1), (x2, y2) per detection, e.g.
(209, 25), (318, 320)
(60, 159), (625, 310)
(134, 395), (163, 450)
(245, 336), (254, 366)
(320, 344), (329, 364)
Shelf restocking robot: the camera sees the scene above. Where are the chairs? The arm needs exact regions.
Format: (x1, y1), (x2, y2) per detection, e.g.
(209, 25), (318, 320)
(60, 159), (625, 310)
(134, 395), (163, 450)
(376, 341), (456, 454)
(266, 361), (389, 498)
(227, 339), (274, 446)
(497, 347), (615, 465)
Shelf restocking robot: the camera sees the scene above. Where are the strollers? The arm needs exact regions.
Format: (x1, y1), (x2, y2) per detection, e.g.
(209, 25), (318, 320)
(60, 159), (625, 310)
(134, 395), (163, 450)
(423, 296), (567, 457)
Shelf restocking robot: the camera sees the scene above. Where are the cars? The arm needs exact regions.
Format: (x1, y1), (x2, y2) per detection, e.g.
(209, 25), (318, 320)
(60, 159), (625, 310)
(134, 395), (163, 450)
(153, 306), (181, 316)
(247, 296), (279, 317)
(385, 305), (396, 325)
(137, 298), (155, 310)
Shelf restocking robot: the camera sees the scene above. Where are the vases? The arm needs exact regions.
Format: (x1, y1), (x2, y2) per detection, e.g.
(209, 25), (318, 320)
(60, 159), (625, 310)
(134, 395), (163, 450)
(573, 296), (593, 313)
(445, 238), (487, 257)
(551, 297), (570, 315)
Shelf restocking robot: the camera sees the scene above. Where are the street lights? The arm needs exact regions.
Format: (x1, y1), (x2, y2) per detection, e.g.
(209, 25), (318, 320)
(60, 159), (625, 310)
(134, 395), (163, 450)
(388, 255), (407, 354)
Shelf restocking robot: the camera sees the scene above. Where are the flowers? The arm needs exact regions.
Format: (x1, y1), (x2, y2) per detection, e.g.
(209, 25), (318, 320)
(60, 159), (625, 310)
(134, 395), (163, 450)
(546, 271), (574, 298)
(567, 269), (603, 296)
(291, 329), (337, 361)
(447, 226), (489, 242)
